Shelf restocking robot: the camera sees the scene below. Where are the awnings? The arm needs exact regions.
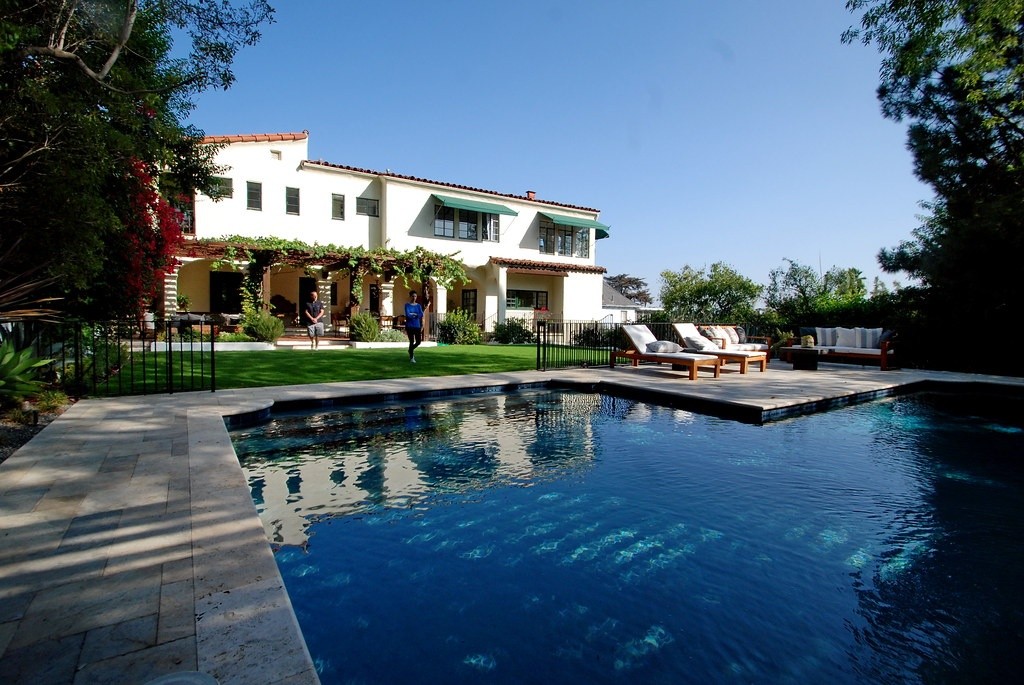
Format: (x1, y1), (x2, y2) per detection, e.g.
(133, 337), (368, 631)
(430, 194), (518, 234)
(537, 211), (609, 246)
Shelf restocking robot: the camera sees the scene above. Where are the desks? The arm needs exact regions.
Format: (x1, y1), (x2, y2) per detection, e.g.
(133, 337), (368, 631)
(780, 347), (829, 371)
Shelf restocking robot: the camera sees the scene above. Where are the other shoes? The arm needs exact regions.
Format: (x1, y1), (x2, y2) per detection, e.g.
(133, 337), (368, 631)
(410, 356), (417, 363)
(311, 348), (316, 351)
(315, 347), (322, 351)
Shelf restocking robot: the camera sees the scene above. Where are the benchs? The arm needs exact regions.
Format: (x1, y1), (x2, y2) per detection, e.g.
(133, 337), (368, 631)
(787, 327), (892, 371)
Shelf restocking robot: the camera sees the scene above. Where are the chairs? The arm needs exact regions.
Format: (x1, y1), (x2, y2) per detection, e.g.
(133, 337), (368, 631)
(332, 314), (349, 337)
(671, 323), (772, 374)
(379, 314), (407, 332)
(610, 325), (720, 380)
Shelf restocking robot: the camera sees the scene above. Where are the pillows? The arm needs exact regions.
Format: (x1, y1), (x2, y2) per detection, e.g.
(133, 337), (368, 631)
(799, 326), (894, 348)
(684, 335), (719, 350)
(704, 325), (747, 345)
(645, 340), (684, 352)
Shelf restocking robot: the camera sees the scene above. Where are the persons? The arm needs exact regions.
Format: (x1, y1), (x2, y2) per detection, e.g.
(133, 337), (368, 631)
(404, 290), (424, 363)
(306, 291), (325, 351)
